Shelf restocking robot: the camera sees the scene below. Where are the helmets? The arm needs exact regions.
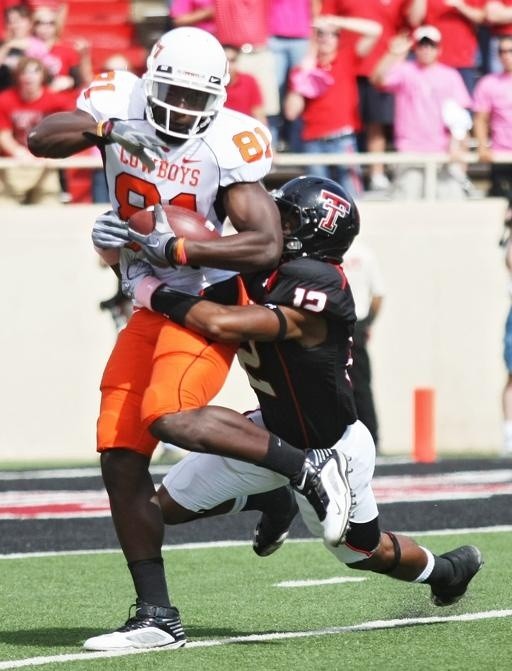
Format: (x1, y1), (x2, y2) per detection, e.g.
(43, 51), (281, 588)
(270, 176), (361, 263)
(144, 27), (230, 138)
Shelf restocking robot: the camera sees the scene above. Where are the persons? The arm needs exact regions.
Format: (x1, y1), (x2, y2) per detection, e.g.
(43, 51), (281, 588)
(119, 175), (482, 606)
(404, 0), (486, 89)
(285, 13), (381, 194)
(503, 203), (512, 454)
(339, 242), (380, 454)
(215, 0), (279, 127)
(170, 0), (218, 33)
(370, 25), (470, 196)
(482, 0), (512, 71)
(1, 59), (66, 205)
(472, 36), (512, 198)
(220, 40), (269, 128)
(28, 27), (354, 650)
(32, 1), (94, 85)
(272, 2), (322, 102)
(318, 1), (406, 191)
(2, 2), (32, 57)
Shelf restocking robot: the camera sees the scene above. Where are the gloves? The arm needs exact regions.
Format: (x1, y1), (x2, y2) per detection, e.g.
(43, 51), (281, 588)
(128, 203), (178, 269)
(92, 210), (128, 251)
(96, 118), (167, 171)
(133, 276), (163, 313)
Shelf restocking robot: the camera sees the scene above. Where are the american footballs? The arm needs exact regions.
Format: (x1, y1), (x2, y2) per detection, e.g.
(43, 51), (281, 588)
(128, 204), (221, 253)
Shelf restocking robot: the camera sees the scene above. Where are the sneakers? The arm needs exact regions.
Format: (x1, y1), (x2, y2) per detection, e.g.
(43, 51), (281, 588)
(295, 448), (356, 547)
(219, 457), (432, 545)
(431, 545), (480, 609)
(251, 488), (300, 557)
(83, 602), (187, 653)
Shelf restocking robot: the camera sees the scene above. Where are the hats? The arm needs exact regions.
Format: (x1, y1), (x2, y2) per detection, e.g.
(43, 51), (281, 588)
(415, 24), (443, 47)
